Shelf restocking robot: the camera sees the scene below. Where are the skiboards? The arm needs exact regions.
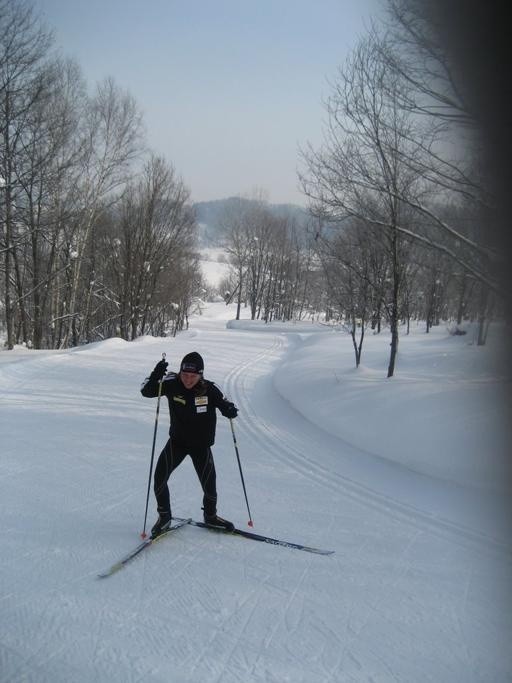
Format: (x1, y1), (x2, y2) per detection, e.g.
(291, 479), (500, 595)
(98, 518), (335, 577)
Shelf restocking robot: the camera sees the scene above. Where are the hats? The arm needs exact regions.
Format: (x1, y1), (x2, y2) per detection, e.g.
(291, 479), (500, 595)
(180, 351), (204, 374)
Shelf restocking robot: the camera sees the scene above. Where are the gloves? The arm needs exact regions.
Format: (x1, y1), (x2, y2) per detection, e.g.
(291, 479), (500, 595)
(154, 359), (168, 380)
(221, 400), (237, 418)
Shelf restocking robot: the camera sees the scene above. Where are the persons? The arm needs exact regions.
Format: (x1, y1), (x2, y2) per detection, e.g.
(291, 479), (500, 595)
(140, 352), (238, 533)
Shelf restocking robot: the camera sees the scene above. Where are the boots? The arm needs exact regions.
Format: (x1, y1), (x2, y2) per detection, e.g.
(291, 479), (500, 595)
(203, 510), (234, 528)
(151, 507), (173, 535)
